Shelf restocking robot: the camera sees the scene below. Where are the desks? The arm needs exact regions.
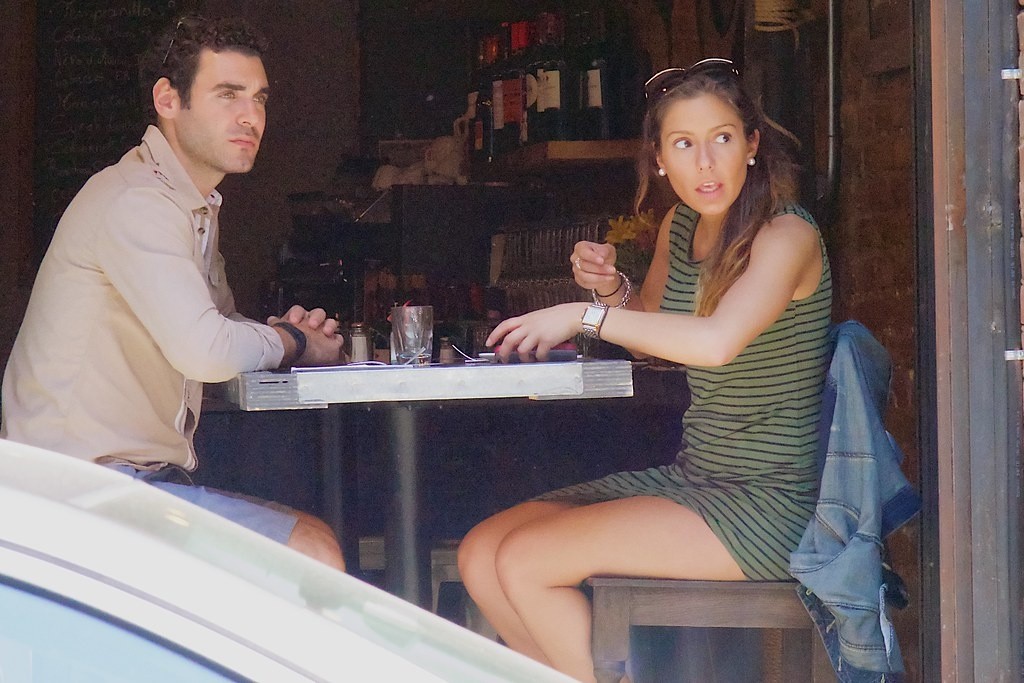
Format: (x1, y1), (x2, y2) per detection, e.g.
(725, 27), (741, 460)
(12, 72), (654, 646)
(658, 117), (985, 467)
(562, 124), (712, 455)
(203, 355), (633, 613)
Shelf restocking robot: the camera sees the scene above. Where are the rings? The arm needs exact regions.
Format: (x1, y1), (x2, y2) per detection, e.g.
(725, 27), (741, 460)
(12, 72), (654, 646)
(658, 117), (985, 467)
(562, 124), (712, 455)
(575, 257), (580, 269)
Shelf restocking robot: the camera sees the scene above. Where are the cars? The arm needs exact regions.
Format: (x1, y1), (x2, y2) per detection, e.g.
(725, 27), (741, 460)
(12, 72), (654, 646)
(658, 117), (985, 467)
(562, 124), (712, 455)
(0, 433), (588, 683)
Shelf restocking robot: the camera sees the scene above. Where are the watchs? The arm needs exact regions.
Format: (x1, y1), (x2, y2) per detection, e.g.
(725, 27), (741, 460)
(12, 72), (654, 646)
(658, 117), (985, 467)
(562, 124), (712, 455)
(580, 301), (610, 340)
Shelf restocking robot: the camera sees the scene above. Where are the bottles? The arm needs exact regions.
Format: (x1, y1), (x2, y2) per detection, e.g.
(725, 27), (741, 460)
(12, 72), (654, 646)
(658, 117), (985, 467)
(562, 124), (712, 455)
(439, 337), (454, 363)
(568, 8), (652, 141)
(348, 322), (372, 363)
(528, 12), (573, 142)
(474, 36), (499, 163)
(500, 21), (528, 149)
(467, 18), (481, 120)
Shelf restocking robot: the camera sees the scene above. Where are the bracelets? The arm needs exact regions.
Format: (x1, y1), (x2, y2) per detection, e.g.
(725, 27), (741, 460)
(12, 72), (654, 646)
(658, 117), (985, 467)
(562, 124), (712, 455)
(274, 322), (306, 366)
(592, 271), (631, 309)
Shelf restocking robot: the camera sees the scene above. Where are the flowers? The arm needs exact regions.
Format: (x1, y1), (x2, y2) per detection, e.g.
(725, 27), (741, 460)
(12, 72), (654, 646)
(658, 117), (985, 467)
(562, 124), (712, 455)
(605, 209), (661, 253)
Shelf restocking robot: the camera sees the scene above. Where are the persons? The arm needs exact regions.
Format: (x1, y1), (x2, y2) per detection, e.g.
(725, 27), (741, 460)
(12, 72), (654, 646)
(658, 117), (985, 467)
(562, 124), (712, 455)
(457, 57), (833, 683)
(0, 10), (346, 573)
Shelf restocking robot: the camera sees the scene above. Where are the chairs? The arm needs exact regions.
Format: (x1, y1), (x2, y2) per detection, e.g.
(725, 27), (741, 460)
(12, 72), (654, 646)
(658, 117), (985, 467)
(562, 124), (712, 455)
(583, 321), (863, 683)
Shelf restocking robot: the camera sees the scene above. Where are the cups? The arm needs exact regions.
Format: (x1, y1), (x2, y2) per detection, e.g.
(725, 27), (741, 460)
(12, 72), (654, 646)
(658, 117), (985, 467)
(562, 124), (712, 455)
(390, 306), (433, 365)
(471, 327), (496, 358)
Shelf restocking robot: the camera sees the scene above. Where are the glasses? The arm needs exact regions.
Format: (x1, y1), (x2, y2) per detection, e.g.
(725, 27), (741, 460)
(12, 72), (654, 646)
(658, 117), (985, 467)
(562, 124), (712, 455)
(161, 17), (209, 66)
(645, 58), (737, 115)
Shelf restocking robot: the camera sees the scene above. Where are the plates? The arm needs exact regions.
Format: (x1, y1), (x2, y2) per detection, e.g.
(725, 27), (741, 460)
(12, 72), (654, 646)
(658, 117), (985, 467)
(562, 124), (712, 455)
(478, 353), (495, 358)
